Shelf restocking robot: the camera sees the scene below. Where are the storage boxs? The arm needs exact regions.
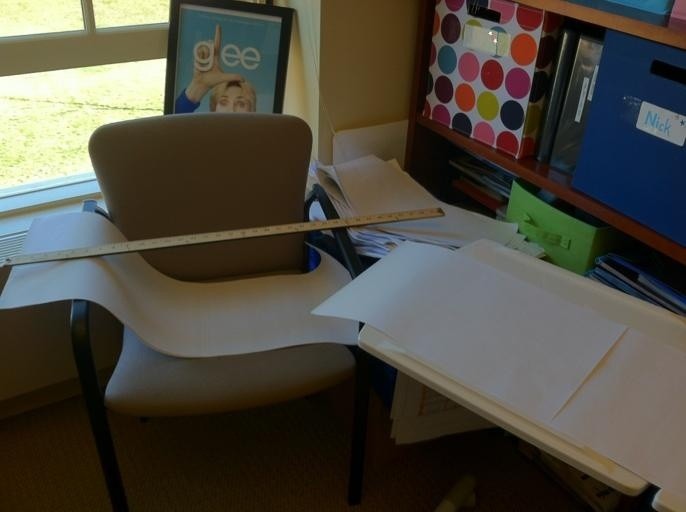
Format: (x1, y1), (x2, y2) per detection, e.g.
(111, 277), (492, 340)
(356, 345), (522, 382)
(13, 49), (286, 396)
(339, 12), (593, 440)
(570, 29), (686, 246)
(421, 0), (561, 160)
(504, 177), (629, 275)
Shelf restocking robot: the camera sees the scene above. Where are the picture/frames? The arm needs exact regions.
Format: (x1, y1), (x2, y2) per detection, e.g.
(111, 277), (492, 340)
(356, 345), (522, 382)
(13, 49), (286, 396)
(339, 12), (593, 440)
(164, 0), (294, 114)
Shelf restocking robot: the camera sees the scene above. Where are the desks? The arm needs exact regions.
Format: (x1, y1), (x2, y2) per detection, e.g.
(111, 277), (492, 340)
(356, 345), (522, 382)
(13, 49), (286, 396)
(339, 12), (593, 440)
(358, 242), (686, 512)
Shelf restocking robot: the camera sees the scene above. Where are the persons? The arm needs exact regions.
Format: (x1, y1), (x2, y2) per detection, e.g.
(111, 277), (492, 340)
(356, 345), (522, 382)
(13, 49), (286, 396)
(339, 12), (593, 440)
(174, 26), (256, 117)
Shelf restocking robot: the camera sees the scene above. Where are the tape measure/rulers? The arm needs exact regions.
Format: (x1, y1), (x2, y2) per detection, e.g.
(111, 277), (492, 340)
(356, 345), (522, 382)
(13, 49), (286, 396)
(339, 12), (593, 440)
(4, 208), (446, 266)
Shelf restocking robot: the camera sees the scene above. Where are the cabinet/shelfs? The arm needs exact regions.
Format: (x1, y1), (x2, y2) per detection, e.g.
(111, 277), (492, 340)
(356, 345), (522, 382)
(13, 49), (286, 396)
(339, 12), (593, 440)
(407, 0), (686, 264)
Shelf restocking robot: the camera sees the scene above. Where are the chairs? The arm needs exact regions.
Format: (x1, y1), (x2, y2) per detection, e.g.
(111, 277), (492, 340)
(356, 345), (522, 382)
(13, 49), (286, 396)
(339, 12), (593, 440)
(70, 113), (372, 512)
